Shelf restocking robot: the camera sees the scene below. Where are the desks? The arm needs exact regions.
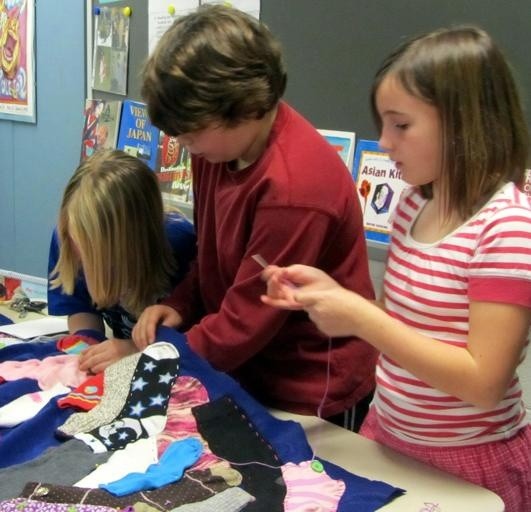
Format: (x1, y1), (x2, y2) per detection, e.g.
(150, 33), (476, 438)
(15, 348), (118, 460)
(268, 406), (505, 512)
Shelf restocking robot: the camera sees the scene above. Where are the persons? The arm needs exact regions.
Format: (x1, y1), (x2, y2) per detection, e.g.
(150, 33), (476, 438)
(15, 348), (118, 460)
(47, 148), (194, 375)
(259, 25), (530, 511)
(131, 1), (377, 431)
